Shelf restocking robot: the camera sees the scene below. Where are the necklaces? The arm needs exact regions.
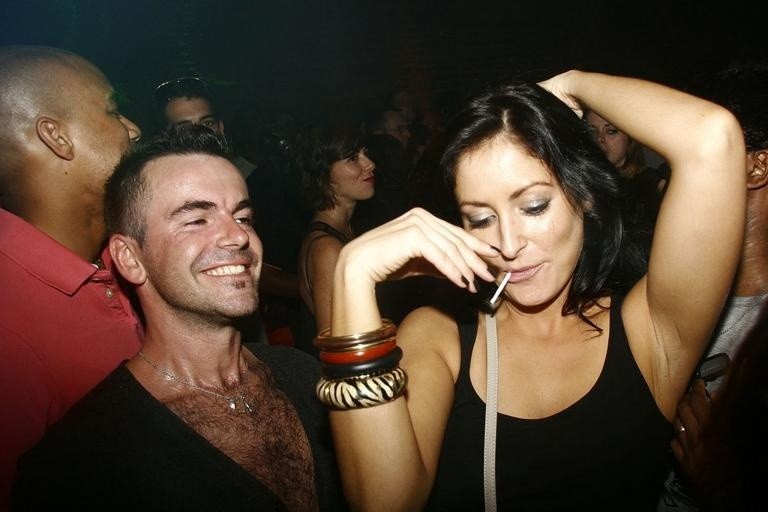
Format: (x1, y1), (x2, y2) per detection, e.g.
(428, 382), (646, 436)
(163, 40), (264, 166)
(139, 350), (254, 414)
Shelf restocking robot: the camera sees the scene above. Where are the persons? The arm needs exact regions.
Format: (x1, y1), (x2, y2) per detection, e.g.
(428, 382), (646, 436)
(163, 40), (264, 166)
(14, 119), (346, 512)
(2, 43), (144, 507)
(314, 69), (747, 511)
(587, 109), (768, 510)
(160, 73), (461, 355)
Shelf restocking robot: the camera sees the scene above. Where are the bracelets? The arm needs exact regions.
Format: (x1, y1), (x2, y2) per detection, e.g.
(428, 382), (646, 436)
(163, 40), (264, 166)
(316, 317), (408, 409)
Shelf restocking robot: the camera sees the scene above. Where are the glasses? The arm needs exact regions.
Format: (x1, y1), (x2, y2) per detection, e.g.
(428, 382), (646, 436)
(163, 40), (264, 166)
(154, 77), (209, 94)
